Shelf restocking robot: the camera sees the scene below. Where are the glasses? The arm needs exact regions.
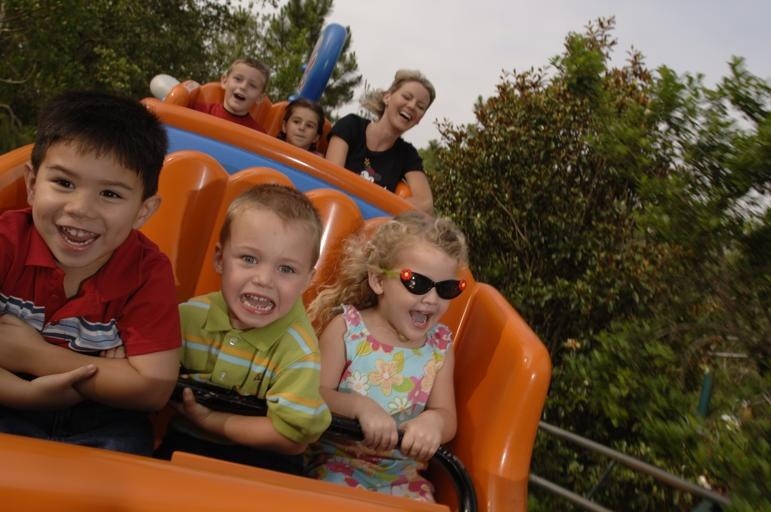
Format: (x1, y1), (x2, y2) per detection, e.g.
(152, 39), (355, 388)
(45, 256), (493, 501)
(383, 269), (469, 300)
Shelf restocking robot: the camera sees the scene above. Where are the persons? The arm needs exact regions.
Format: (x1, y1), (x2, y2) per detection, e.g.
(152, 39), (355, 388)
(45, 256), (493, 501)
(190, 56), (269, 134)
(302, 212), (468, 503)
(276, 98), (324, 158)
(155, 183), (333, 479)
(2, 87), (182, 455)
(323, 69), (436, 215)
